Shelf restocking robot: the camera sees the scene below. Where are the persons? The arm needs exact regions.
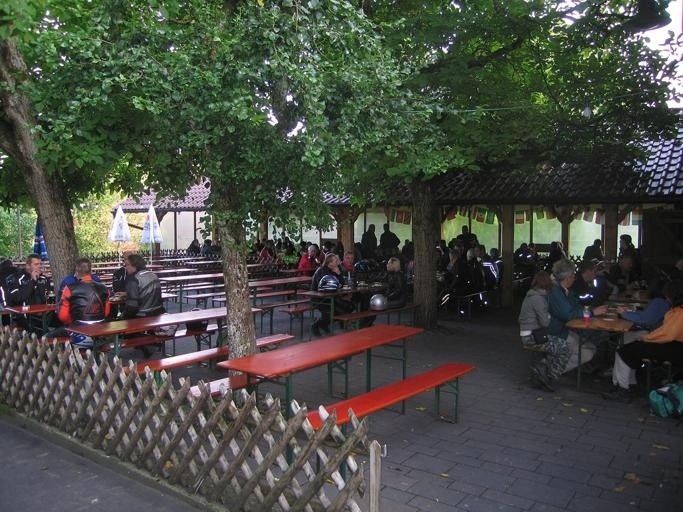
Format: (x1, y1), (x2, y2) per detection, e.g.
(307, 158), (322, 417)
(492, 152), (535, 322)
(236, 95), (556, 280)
(4, 254), (51, 330)
(183, 223), (414, 338)
(43, 257), (111, 355)
(56, 256), (102, 302)
(114, 254), (165, 360)
(0, 258), (19, 326)
(112, 251), (136, 313)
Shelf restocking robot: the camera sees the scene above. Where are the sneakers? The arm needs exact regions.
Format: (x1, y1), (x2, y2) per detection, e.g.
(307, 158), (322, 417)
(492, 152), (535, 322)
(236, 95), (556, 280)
(602, 384), (637, 404)
(311, 320), (329, 336)
(528, 363), (555, 392)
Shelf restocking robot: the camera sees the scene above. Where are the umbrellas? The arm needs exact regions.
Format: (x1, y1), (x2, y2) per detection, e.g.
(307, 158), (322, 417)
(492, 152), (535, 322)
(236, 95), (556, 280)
(109, 204), (130, 259)
(32, 216), (50, 267)
(138, 203), (163, 271)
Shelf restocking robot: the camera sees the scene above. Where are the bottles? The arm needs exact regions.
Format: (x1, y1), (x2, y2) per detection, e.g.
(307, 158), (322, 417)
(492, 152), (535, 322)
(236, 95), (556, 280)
(583, 306), (591, 328)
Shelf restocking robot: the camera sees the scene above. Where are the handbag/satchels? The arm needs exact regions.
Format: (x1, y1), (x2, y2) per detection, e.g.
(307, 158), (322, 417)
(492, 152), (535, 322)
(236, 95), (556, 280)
(533, 329), (547, 344)
(648, 381), (683, 418)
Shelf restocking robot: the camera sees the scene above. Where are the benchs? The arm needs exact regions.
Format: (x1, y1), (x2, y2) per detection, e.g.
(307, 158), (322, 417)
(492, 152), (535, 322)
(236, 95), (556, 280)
(298, 359), (477, 458)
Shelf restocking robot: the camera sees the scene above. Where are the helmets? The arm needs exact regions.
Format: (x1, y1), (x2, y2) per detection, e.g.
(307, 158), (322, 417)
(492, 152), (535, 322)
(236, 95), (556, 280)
(369, 294), (389, 311)
(317, 274), (339, 291)
(154, 326), (178, 337)
(186, 308), (208, 332)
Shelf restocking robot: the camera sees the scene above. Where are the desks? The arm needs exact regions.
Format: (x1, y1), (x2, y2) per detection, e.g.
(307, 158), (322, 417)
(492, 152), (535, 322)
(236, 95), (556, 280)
(0, 255), (534, 397)
(521, 256), (682, 404)
